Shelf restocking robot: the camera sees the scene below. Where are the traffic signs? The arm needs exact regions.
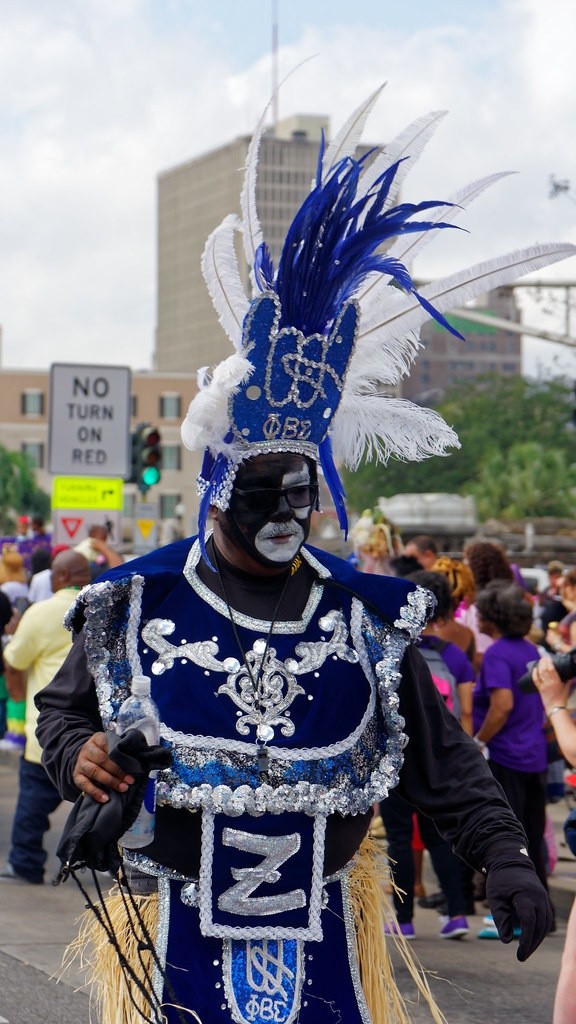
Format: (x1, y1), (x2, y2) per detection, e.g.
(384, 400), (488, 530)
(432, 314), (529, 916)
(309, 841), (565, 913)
(51, 477), (125, 509)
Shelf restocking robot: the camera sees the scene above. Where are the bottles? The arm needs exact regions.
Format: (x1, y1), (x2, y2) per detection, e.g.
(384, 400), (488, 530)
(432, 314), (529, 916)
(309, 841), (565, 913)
(117, 676), (160, 848)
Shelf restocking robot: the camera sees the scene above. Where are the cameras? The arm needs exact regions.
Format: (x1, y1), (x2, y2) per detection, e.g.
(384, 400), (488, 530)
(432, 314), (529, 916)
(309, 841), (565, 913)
(517, 646), (576, 695)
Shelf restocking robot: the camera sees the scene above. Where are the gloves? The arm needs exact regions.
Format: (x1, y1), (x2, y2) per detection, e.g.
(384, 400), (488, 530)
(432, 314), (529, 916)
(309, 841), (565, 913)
(472, 839), (552, 963)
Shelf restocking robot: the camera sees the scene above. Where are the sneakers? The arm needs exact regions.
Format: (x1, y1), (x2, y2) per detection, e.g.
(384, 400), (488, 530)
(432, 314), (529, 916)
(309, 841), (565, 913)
(383, 920), (415, 940)
(439, 913), (470, 939)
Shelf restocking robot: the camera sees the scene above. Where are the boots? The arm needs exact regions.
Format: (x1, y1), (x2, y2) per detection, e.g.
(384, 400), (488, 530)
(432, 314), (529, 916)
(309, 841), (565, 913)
(383, 848), (425, 898)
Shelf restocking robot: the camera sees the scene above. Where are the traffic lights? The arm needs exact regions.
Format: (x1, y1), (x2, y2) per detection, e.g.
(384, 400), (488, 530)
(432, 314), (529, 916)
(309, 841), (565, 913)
(123, 422), (163, 491)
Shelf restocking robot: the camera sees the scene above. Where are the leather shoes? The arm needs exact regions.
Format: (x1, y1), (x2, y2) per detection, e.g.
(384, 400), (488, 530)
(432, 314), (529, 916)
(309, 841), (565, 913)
(434, 901), (476, 916)
(417, 889), (448, 907)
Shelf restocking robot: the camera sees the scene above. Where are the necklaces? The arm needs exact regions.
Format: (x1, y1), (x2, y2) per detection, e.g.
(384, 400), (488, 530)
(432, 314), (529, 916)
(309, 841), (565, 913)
(211, 534), (293, 773)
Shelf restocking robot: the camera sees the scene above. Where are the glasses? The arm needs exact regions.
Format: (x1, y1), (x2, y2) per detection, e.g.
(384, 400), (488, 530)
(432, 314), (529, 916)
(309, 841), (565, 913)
(228, 481), (325, 516)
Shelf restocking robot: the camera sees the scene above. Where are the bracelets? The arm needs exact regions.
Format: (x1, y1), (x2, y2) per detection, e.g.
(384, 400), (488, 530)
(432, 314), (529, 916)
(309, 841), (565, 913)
(549, 706), (566, 718)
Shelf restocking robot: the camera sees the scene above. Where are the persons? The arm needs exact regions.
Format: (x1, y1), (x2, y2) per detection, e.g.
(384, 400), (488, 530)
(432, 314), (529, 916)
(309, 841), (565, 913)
(351, 513), (576, 1024)
(33, 451), (554, 1024)
(0, 519), (123, 883)
